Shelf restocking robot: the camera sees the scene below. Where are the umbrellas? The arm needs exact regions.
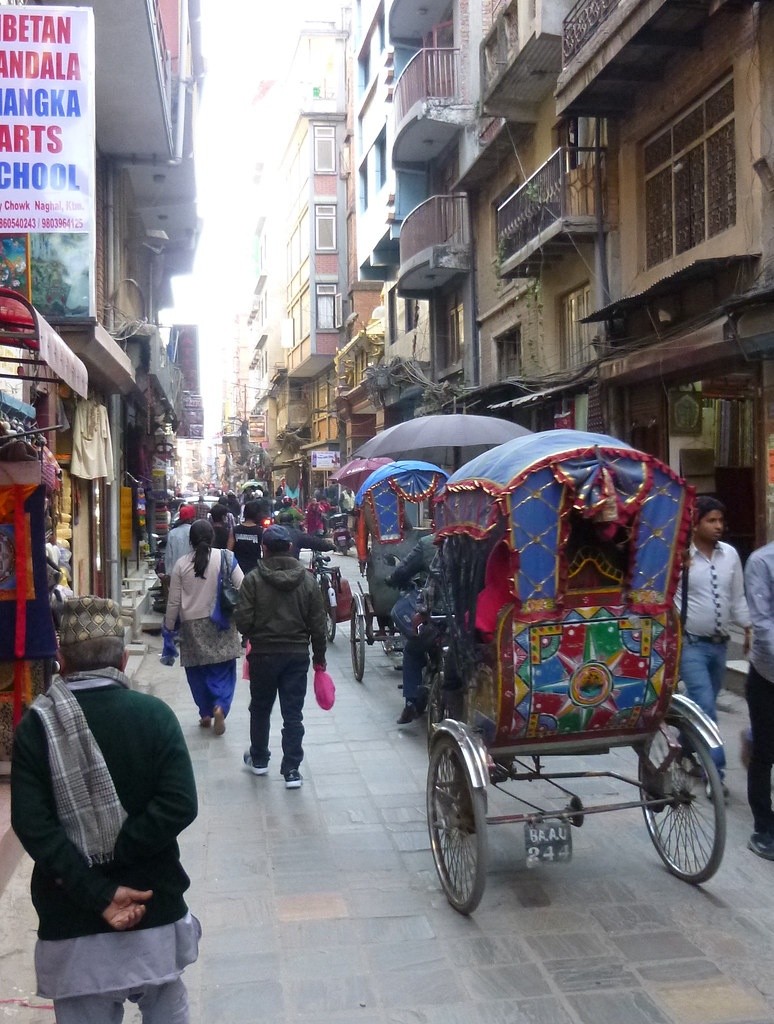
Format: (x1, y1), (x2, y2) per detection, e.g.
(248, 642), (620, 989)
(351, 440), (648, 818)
(348, 414), (534, 459)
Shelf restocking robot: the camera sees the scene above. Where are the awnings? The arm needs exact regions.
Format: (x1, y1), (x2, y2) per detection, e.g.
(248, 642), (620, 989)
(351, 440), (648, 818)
(574, 253), (762, 325)
(0, 287), (88, 400)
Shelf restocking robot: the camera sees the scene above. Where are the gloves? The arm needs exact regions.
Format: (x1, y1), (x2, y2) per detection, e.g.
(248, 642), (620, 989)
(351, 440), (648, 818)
(332, 543), (337, 553)
(384, 576), (398, 589)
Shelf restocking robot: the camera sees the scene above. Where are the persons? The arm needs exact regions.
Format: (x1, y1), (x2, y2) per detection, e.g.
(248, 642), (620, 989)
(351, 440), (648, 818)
(672, 496), (751, 800)
(227, 501), (266, 647)
(276, 484), (283, 496)
(210, 504), (230, 549)
(165, 505), (197, 582)
(339, 486), (356, 527)
(742, 540), (774, 860)
(279, 512), (337, 562)
(233, 524), (327, 787)
(11, 597), (202, 1024)
(195, 496), (210, 520)
(165, 519), (244, 735)
(306, 497), (332, 535)
(384, 533), (454, 724)
(247, 485), (273, 513)
(219, 490), (245, 527)
(274, 494), (305, 528)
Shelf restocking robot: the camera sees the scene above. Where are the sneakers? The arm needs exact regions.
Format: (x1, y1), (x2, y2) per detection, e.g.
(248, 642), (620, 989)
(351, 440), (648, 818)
(244, 753), (268, 774)
(285, 769), (303, 788)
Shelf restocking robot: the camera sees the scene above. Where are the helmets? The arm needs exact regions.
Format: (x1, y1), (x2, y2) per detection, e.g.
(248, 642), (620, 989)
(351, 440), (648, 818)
(281, 496), (292, 506)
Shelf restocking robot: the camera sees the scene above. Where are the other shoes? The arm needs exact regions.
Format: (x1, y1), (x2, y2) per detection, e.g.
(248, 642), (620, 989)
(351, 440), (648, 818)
(213, 706), (225, 735)
(705, 782), (728, 799)
(678, 750), (703, 776)
(748, 831), (774, 860)
(397, 701), (419, 723)
(200, 716), (212, 728)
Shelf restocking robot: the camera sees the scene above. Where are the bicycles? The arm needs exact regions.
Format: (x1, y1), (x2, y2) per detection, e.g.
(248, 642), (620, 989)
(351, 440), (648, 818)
(302, 546), (345, 642)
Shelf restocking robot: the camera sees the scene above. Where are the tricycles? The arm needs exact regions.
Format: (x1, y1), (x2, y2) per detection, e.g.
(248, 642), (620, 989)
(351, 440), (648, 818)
(391, 428), (729, 918)
(347, 413), (543, 685)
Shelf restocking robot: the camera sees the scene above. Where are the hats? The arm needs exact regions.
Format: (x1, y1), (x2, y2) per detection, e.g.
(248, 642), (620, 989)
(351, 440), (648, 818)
(181, 506), (196, 520)
(252, 489), (263, 499)
(59, 595), (125, 645)
(263, 525), (292, 546)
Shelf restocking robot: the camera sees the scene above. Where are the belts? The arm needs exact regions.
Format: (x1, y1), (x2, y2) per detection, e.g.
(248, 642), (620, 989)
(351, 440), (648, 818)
(698, 636), (730, 644)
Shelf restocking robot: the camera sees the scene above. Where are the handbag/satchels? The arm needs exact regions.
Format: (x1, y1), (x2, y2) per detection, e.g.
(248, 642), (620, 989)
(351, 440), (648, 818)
(242, 640), (251, 680)
(391, 589), (425, 640)
(315, 666), (335, 710)
(221, 550), (238, 607)
(210, 553), (240, 631)
(325, 567), (354, 624)
